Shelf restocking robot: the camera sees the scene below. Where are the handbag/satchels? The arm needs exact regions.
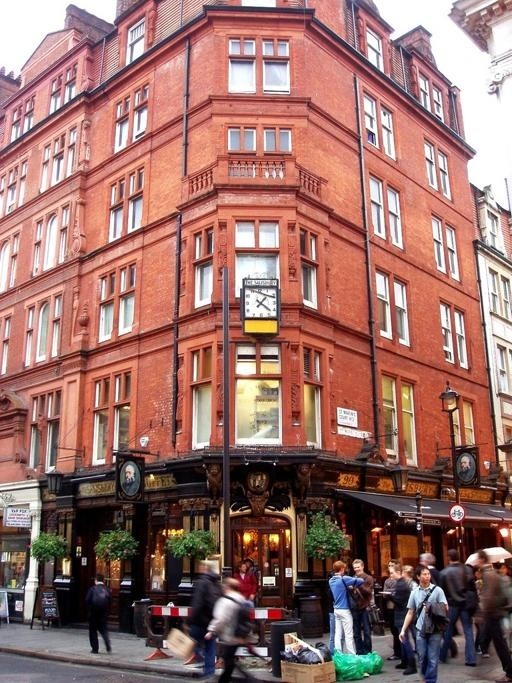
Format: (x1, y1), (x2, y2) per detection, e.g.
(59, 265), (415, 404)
(367, 605), (384, 627)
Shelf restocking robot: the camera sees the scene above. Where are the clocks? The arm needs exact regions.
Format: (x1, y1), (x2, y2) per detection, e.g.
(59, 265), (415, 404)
(242, 285), (279, 320)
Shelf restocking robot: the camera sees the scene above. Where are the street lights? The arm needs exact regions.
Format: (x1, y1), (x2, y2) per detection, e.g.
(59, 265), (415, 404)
(439, 377), (465, 569)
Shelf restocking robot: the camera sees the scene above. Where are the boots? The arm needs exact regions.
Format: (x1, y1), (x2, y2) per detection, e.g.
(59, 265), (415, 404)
(402, 654), (418, 676)
(394, 654), (408, 669)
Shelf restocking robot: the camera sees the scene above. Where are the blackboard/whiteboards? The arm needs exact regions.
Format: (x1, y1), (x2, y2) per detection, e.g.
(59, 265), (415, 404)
(39, 591), (59, 619)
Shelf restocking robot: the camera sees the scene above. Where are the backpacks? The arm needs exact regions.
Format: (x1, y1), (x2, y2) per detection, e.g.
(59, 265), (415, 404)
(221, 593), (253, 640)
(91, 585), (111, 607)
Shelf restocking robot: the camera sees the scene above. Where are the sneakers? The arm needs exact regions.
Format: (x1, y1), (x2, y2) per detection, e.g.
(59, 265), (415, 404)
(474, 647), (490, 658)
(386, 654), (401, 661)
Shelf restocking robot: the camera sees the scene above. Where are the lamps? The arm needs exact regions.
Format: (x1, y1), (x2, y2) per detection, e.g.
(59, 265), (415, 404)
(389, 461), (412, 493)
(44, 464), (66, 495)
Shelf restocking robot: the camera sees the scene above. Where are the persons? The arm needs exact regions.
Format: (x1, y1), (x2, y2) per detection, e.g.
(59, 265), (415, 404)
(457, 456), (475, 481)
(295, 463), (315, 501)
(121, 464), (139, 495)
(201, 463), (222, 506)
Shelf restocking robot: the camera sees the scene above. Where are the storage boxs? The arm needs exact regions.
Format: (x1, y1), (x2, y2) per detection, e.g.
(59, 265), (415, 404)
(278, 656), (338, 682)
